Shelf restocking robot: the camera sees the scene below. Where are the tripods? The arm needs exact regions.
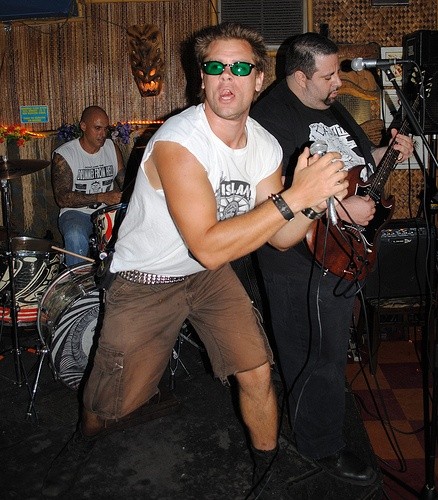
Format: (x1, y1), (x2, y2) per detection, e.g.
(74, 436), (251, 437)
(0, 180), (49, 388)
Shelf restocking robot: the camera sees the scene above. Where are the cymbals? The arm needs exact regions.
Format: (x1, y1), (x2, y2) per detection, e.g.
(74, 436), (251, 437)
(0, 158), (50, 180)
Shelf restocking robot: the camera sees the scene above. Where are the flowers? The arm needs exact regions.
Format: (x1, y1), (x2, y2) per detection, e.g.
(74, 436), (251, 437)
(57, 122), (82, 146)
(0, 124), (32, 148)
(109, 122), (133, 145)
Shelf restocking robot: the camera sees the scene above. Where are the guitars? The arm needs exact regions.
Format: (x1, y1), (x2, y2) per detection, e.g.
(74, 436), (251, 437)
(305, 67), (434, 282)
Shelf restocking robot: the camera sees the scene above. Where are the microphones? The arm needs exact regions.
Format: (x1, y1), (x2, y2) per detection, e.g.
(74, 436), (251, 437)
(310, 139), (340, 224)
(351, 58), (413, 71)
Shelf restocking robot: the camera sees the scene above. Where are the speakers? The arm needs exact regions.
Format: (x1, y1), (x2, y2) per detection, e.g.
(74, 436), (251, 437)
(360, 217), (438, 299)
(401, 30), (437, 135)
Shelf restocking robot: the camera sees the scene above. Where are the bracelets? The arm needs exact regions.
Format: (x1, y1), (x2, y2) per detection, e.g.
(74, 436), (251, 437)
(271, 195), (295, 222)
(301, 208), (328, 220)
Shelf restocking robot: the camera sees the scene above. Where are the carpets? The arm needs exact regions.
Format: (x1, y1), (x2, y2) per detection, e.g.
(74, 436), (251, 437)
(0, 318), (387, 500)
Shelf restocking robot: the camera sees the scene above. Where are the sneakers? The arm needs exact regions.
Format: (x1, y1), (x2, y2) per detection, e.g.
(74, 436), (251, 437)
(40, 427), (98, 500)
(245, 440), (290, 500)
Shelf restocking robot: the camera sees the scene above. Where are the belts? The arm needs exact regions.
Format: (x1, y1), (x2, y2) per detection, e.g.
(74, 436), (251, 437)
(117, 270), (186, 285)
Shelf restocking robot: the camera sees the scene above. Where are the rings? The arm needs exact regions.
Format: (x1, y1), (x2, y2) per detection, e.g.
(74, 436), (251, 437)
(398, 156), (403, 161)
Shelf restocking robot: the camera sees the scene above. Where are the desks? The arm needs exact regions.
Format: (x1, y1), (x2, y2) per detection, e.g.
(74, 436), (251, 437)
(368, 296), (426, 376)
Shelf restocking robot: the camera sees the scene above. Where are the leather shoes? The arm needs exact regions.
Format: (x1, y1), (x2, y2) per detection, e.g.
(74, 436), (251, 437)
(315, 449), (377, 486)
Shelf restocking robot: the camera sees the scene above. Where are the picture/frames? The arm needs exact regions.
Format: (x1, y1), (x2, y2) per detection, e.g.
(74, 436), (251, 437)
(382, 89), (402, 128)
(20, 105), (49, 123)
(381, 47), (403, 86)
(394, 133), (428, 169)
(371, 0), (412, 7)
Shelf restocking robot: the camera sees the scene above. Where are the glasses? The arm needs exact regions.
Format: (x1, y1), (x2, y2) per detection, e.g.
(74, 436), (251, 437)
(200, 61), (258, 76)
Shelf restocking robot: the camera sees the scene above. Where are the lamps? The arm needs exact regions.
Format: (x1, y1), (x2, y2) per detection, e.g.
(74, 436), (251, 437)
(126, 24), (164, 97)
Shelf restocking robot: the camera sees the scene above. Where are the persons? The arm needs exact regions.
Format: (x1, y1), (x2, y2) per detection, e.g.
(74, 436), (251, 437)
(249, 32), (413, 485)
(51, 105), (126, 266)
(45, 21), (349, 500)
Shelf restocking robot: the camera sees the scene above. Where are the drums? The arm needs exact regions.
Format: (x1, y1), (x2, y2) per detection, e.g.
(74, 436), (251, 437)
(0, 235), (66, 326)
(35, 263), (102, 391)
(89, 203), (129, 259)
